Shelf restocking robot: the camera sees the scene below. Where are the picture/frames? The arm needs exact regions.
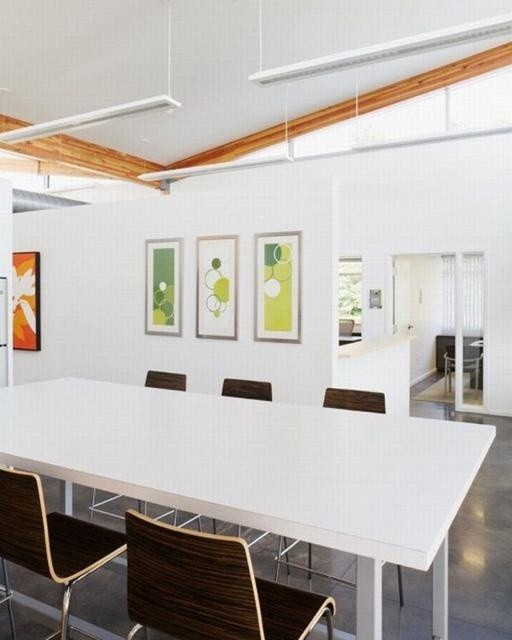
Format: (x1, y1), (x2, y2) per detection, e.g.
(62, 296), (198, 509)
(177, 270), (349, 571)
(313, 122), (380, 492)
(196, 234), (239, 340)
(12, 251), (42, 353)
(143, 237), (184, 337)
(253, 229), (301, 344)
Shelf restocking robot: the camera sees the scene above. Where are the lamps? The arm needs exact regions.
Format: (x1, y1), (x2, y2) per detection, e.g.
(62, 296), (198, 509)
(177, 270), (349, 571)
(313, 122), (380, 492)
(139, 127), (512, 180)
(247, 14), (511, 86)
(0, 95), (181, 142)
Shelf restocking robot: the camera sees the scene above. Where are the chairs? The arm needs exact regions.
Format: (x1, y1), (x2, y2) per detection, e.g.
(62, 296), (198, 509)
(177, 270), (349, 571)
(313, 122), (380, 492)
(123, 508), (337, 640)
(0, 469), (145, 640)
(274, 386), (408, 607)
(443, 344), (483, 394)
(87, 370), (203, 538)
(170, 378), (291, 576)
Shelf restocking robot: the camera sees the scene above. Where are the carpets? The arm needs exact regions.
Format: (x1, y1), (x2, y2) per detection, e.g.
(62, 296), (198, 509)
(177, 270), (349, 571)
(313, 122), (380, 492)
(413, 371), (485, 406)
